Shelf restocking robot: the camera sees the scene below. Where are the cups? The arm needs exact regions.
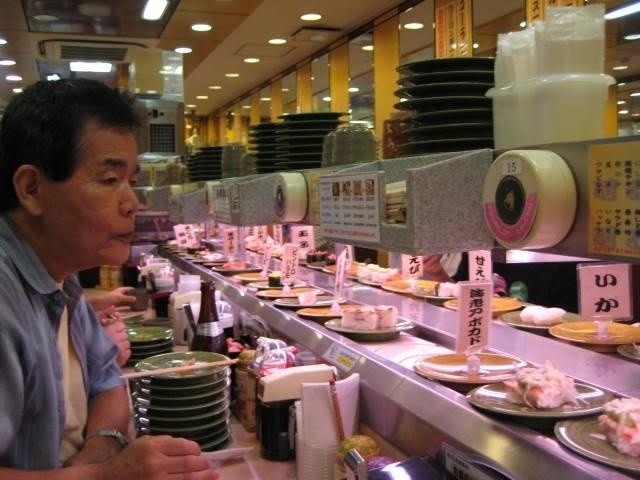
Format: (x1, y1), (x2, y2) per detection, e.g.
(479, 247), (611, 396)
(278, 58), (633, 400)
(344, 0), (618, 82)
(127, 287), (149, 312)
(484, 70), (618, 151)
(148, 259), (168, 277)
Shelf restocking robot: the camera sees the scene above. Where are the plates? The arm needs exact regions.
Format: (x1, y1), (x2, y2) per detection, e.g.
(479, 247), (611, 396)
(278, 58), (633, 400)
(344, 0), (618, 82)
(130, 348), (237, 456)
(186, 146), (223, 182)
(412, 351), (639, 477)
(156, 229), (640, 361)
(390, 56), (497, 158)
(121, 315), (175, 368)
(246, 111), (353, 174)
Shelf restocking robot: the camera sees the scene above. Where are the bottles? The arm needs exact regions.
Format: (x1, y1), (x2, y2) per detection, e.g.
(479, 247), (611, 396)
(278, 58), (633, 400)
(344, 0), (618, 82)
(230, 347), (403, 480)
(318, 119), (377, 167)
(136, 155), (187, 187)
(188, 281), (228, 358)
(98, 263), (122, 290)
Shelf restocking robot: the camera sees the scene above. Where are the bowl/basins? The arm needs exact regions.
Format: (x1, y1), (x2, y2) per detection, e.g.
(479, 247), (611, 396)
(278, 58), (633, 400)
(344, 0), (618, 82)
(220, 143), (258, 178)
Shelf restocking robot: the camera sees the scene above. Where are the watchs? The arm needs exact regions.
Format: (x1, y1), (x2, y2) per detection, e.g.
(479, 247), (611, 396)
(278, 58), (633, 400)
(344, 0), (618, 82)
(85, 428), (129, 448)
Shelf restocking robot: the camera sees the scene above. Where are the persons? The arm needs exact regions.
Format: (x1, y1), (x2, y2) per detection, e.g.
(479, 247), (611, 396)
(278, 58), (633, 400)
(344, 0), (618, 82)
(0, 78), (222, 480)
(85, 286), (137, 367)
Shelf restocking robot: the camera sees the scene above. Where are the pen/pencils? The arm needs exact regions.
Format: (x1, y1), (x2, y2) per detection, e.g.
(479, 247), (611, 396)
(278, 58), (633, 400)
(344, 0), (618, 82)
(329, 378), (345, 441)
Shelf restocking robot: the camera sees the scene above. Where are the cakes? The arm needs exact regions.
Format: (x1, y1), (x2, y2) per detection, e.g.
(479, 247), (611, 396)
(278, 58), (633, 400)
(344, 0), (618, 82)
(341, 304), (398, 330)
(503, 362), (579, 409)
(599, 397), (640, 458)
